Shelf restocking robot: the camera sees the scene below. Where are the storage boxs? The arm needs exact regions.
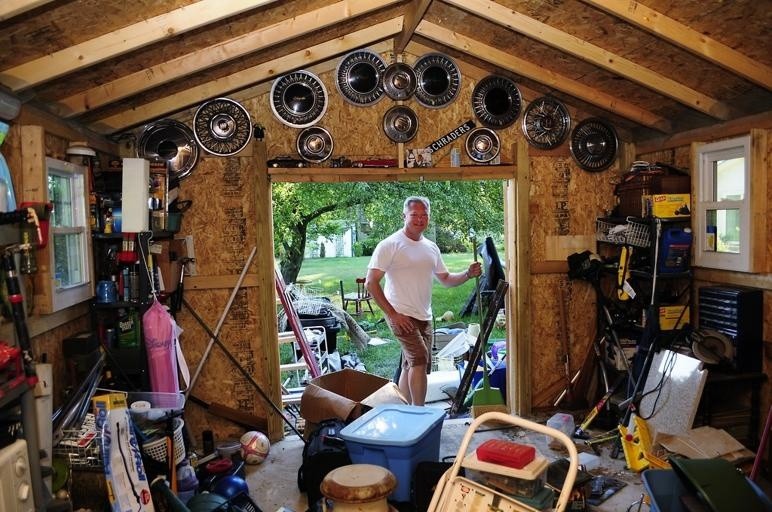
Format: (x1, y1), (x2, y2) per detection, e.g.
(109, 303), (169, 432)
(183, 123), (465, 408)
(614, 172), (688, 219)
(640, 194), (691, 219)
(298, 370), (411, 445)
(642, 467), (772, 512)
(285, 296), (341, 361)
(430, 322), (466, 374)
(641, 305), (691, 329)
(339, 403), (447, 501)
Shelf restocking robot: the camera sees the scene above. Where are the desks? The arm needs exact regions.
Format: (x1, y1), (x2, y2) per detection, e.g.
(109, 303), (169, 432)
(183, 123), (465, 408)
(694, 374), (766, 457)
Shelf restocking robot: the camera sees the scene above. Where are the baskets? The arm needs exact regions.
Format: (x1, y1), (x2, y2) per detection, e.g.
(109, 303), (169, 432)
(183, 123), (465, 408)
(298, 286), (323, 317)
(141, 417), (186, 467)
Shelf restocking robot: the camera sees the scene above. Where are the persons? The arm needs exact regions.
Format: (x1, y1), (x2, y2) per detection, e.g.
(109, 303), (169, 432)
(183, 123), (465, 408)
(363, 196), (482, 406)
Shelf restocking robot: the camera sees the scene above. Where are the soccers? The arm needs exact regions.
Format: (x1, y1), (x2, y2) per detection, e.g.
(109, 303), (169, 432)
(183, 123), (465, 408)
(240, 431), (270, 465)
(444, 310), (454, 321)
(217, 476), (249, 500)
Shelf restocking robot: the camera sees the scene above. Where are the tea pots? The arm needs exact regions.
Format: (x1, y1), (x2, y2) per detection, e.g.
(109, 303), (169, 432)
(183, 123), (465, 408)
(96, 280), (116, 304)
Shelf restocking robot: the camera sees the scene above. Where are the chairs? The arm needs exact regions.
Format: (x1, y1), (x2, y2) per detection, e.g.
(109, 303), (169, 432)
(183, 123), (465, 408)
(343, 278), (375, 317)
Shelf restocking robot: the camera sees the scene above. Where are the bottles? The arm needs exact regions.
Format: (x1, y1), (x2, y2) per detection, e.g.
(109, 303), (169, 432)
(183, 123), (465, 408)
(706, 225), (717, 251)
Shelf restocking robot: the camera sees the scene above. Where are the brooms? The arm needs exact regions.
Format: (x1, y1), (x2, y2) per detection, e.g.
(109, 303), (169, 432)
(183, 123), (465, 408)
(469, 236), (509, 420)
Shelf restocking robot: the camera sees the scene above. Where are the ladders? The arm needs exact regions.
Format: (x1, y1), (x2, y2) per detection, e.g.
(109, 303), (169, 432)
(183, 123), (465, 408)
(425, 411), (579, 512)
(449, 278), (509, 418)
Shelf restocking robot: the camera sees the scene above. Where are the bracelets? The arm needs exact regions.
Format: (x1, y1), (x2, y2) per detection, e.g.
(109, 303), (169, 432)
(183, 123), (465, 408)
(465, 270), (471, 279)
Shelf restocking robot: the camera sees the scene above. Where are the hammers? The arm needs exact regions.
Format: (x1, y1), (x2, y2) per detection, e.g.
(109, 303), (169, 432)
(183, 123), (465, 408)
(175, 257), (195, 311)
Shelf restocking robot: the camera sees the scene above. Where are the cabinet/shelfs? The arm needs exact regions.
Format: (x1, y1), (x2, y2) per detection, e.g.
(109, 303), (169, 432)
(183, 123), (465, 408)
(277, 325), (331, 405)
(595, 218), (695, 433)
(92, 168), (174, 391)
